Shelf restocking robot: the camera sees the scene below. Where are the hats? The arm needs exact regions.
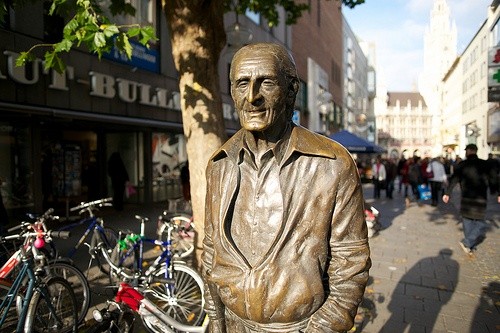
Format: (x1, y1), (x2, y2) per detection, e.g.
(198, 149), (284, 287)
(464, 144), (478, 152)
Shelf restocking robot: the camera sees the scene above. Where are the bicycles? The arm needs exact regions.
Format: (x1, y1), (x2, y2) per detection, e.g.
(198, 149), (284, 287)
(0, 197), (209, 333)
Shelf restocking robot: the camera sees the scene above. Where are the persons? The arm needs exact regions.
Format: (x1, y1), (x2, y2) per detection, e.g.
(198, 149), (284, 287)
(442, 144), (500, 255)
(201, 43), (371, 333)
(354, 151), (500, 207)
(108, 151), (129, 211)
(180, 161), (191, 203)
(11, 177), (31, 221)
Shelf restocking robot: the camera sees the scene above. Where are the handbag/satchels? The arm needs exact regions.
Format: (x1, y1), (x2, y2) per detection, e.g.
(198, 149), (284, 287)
(417, 183), (432, 201)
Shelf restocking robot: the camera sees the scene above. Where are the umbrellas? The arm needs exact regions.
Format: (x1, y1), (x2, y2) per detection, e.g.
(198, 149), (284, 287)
(326, 131), (388, 162)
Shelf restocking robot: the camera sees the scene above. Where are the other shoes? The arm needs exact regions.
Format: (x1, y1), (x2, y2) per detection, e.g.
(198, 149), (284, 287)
(460, 242), (471, 255)
